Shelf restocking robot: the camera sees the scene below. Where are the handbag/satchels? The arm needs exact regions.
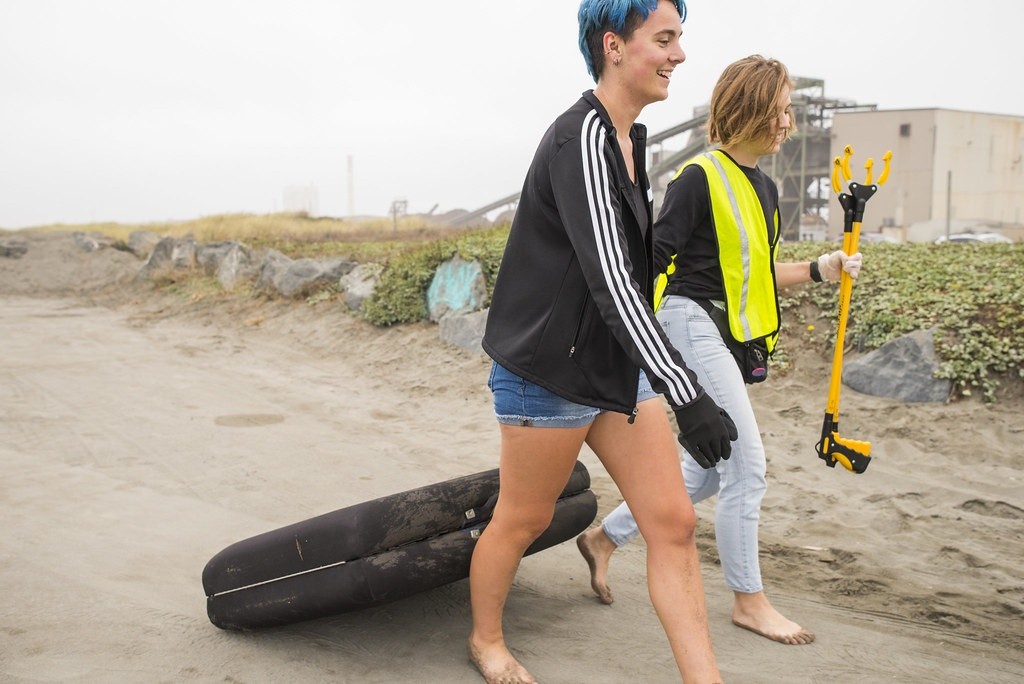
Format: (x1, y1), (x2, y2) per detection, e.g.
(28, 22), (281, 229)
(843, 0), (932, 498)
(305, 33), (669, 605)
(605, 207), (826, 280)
(692, 296), (768, 385)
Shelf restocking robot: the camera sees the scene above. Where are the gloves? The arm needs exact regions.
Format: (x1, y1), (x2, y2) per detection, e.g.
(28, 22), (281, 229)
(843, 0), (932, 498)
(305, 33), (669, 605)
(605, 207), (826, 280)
(675, 391), (738, 469)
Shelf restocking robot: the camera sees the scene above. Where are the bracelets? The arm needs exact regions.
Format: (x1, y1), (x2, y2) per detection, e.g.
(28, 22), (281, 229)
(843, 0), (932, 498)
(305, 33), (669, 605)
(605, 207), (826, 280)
(810, 261), (822, 283)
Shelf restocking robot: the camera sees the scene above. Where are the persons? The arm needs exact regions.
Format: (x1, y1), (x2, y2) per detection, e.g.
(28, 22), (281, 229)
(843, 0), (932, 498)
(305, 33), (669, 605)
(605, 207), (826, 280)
(576, 53), (861, 647)
(468, 0), (737, 683)
